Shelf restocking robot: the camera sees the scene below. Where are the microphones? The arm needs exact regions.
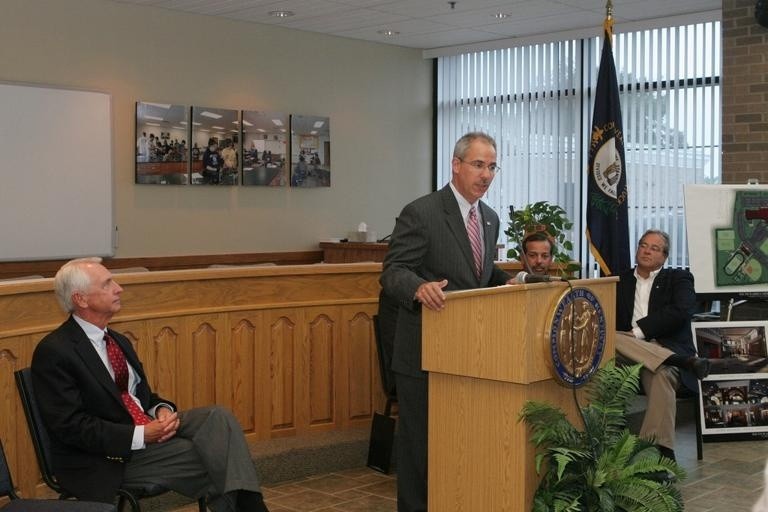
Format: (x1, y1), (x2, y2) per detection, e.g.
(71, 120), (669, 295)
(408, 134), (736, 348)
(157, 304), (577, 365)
(515, 271), (562, 283)
(510, 205), (534, 275)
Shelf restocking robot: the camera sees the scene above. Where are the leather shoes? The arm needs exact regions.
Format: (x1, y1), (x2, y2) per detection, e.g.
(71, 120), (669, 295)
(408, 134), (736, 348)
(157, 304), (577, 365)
(692, 356), (712, 380)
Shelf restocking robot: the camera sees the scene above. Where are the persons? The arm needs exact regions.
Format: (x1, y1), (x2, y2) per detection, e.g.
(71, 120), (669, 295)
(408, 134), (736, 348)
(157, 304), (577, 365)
(615, 230), (711, 476)
(521, 234), (554, 277)
(32, 254), (269, 512)
(378, 130), (521, 512)
(138, 129), (321, 186)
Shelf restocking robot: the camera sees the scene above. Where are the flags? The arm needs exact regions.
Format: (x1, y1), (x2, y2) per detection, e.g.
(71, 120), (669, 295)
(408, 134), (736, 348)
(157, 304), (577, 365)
(584, 14), (634, 287)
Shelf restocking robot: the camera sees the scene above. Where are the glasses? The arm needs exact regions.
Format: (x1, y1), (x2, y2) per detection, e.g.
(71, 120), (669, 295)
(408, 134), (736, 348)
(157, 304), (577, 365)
(458, 157), (500, 174)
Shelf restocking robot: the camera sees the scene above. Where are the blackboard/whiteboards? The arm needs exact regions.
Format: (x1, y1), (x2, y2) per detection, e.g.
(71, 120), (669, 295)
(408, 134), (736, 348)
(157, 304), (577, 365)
(0, 80), (116, 262)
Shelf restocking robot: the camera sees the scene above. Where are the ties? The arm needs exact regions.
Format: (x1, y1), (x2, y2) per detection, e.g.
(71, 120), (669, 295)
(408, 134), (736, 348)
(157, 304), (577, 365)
(103, 331), (154, 425)
(467, 206), (482, 281)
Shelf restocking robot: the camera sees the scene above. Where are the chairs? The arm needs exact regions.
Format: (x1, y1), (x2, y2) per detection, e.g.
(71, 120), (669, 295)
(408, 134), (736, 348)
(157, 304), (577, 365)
(1, 368), (206, 511)
